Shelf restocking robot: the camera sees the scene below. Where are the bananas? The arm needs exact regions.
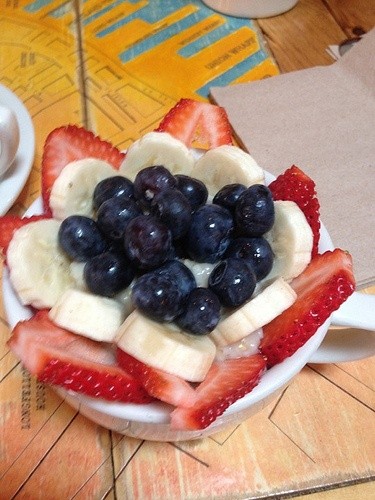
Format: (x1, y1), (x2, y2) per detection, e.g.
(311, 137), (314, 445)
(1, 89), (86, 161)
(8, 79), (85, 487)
(7, 131), (315, 385)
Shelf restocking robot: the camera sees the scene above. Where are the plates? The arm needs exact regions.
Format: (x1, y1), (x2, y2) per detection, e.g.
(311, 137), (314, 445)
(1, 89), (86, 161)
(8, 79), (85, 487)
(0, 80), (37, 218)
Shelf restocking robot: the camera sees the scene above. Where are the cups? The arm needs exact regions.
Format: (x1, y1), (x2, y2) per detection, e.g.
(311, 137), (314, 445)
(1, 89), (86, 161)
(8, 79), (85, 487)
(3, 150), (374, 443)
(201, 0), (298, 18)
(0, 103), (20, 174)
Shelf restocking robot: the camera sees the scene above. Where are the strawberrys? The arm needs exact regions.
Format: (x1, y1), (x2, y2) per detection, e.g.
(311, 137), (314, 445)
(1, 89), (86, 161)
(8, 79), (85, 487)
(7, 311), (267, 433)
(0, 98), (233, 314)
(258, 163), (355, 371)
